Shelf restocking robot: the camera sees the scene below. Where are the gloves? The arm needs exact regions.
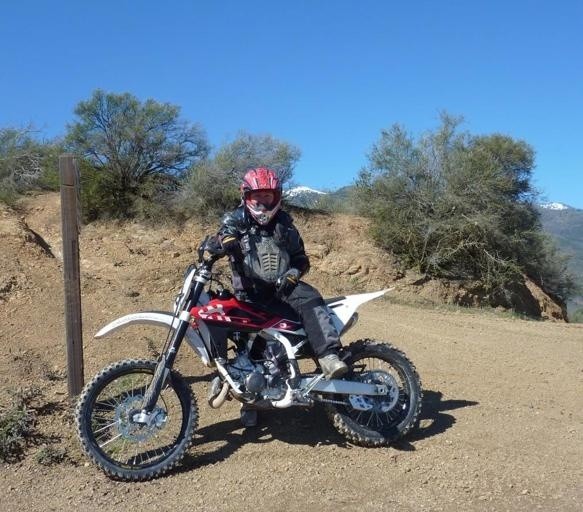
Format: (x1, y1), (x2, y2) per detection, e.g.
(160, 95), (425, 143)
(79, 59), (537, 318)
(221, 235), (244, 257)
(276, 268), (301, 295)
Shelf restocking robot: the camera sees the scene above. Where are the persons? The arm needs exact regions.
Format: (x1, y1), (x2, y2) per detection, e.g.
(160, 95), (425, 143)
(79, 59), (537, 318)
(218, 166), (350, 428)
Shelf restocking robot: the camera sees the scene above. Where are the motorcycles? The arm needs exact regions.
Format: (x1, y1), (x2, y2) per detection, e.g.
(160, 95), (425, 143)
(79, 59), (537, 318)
(75, 232), (425, 485)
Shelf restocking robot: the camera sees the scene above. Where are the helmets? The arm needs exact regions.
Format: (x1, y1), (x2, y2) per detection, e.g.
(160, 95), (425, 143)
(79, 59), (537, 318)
(240, 168), (281, 209)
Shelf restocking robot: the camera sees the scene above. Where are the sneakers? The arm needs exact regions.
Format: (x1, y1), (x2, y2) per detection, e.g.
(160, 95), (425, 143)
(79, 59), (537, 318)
(318, 353), (348, 381)
(240, 403), (257, 427)
